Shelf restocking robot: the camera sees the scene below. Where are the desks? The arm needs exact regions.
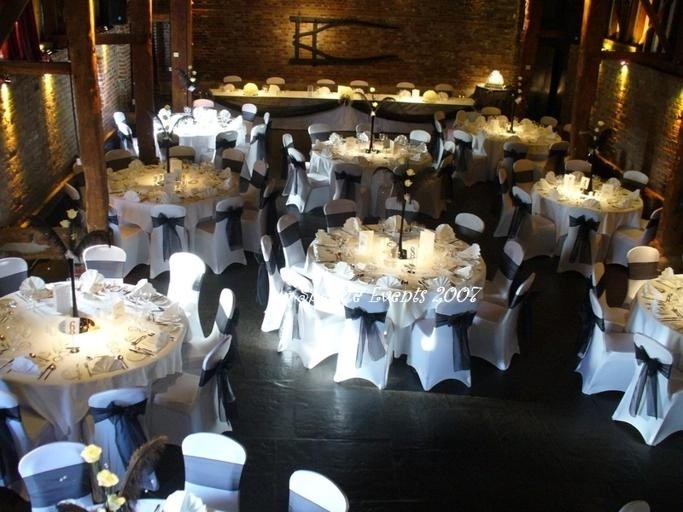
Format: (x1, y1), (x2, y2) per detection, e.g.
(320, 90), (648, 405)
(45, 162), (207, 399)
(470, 120), (562, 159)
(0, 278), (188, 442)
(306, 222), (487, 359)
(631, 273), (682, 361)
(310, 136), (433, 204)
(157, 116), (242, 160)
(48, 498), (230, 512)
(531, 173), (644, 235)
(106, 163), (241, 227)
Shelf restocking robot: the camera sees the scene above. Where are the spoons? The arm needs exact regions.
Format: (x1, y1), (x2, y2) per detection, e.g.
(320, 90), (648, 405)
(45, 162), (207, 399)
(29, 352), (49, 362)
(44, 364), (56, 380)
(118, 354), (129, 369)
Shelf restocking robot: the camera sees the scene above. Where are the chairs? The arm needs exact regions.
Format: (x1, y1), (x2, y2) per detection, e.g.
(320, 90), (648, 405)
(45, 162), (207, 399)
(621, 170), (649, 192)
(575, 288), (635, 395)
(350, 80), (368, 94)
(148, 334), (232, 439)
(118, 122), (139, 158)
(194, 196), (247, 274)
(238, 159), (269, 210)
(192, 99), (214, 111)
(453, 213), (486, 244)
(435, 119), (444, 168)
(108, 203), (149, 277)
(179, 432), (248, 512)
(355, 123), (377, 140)
(286, 463), (353, 512)
(72, 159), (85, 194)
(484, 240), (525, 306)
(0, 390), (53, 481)
(149, 204), (188, 279)
(156, 132), (179, 164)
(333, 292), (396, 390)
(264, 111), (272, 148)
(508, 186), (555, 259)
(241, 178), (277, 254)
(406, 297), (478, 391)
(221, 148), (245, 175)
(0, 257), (28, 298)
(105, 149), (132, 171)
(440, 140), (456, 165)
(307, 123), (331, 144)
(539, 141), (569, 177)
(611, 333), (683, 446)
(557, 207), (602, 276)
(396, 82), (416, 94)
(17, 441), (88, 512)
(83, 244), (127, 284)
(275, 267), (337, 369)
(435, 84), (454, 98)
(513, 159), (534, 193)
(625, 246), (659, 305)
(433, 111), (445, 121)
(260, 234), (305, 332)
(541, 116), (558, 127)
(610, 207), (664, 264)
(467, 271), (536, 370)
(415, 154), (454, 217)
(287, 147), (331, 215)
(481, 106), (502, 120)
(167, 146), (197, 172)
(222, 74), (242, 87)
(182, 287), (238, 369)
(494, 168), (517, 237)
(281, 133), (310, 196)
(591, 262), (631, 333)
(167, 251), (206, 326)
(213, 131), (238, 168)
(266, 76), (285, 89)
(276, 214), (306, 268)
(329, 163), (368, 221)
(323, 199), (356, 233)
(316, 79), (338, 92)
(453, 130), (486, 184)
(88, 387), (148, 483)
(503, 142), (529, 161)
(409, 130), (431, 148)
(113, 112), (138, 145)
(245, 125), (267, 174)
(561, 124), (571, 139)
(564, 160), (592, 177)
(241, 103), (258, 126)
(65, 181), (82, 211)
(385, 197), (420, 224)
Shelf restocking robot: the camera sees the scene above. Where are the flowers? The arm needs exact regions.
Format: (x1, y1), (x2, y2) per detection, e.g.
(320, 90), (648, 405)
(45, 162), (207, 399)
(51, 208), (87, 259)
(371, 157), (435, 204)
(81, 444), (127, 512)
(339, 86), (396, 118)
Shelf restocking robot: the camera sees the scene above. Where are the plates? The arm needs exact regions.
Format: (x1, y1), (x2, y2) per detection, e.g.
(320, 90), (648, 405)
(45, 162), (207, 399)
(127, 351), (146, 362)
(62, 368), (80, 380)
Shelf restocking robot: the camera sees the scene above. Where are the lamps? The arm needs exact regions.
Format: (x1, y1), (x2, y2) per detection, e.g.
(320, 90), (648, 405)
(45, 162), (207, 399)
(169, 52), (198, 92)
(486, 68), (505, 88)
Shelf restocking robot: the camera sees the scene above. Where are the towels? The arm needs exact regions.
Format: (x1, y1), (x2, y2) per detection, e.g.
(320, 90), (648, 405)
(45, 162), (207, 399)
(196, 186), (218, 199)
(631, 189), (641, 201)
(156, 302), (181, 324)
(582, 198), (602, 209)
(330, 260), (358, 282)
(78, 268), (104, 293)
(460, 242), (482, 259)
(162, 489), (206, 511)
(130, 278), (159, 301)
(385, 215), (409, 232)
(433, 224), (455, 244)
(345, 216), (362, 234)
(122, 189), (144, 203)
(425, 275), (453, 292)
(89, 355), (122, 373)
(19, 274), (47, 295)
(663, 266), (677, 289)
(221, 176), (234, 191)
(454, 261), (477, 279)
(220, 166), (233, 179)
(9, 355), (41, 374)
(616, 196), (633, 207)
(313, 229), (338, 263)
(375, 274), (403, 290)
